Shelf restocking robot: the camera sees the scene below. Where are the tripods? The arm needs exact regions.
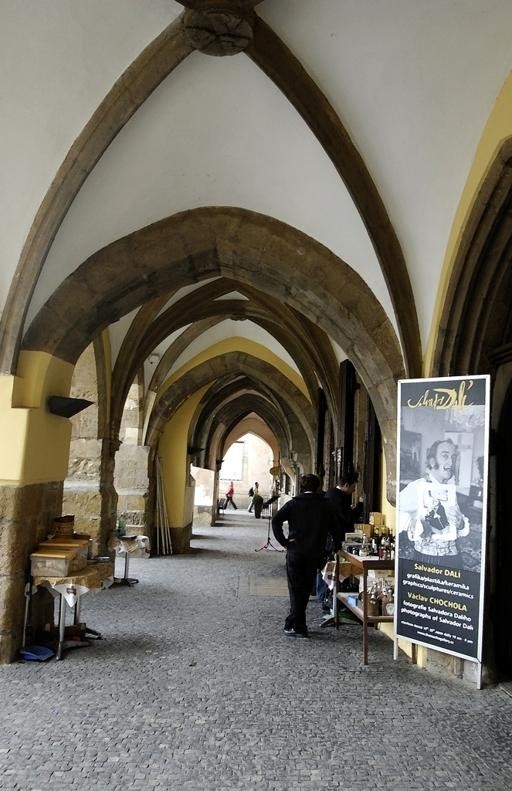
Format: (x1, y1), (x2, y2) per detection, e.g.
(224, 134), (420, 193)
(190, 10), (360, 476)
(254, 505), (284, 552)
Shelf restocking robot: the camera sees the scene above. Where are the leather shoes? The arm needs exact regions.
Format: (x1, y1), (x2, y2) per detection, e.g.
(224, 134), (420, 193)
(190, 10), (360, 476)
(283, 627), (304, 638)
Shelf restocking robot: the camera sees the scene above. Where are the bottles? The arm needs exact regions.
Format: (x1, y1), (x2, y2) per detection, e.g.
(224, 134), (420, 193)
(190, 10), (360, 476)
(370, 576), (395, 604)
(347, 526), (397, 559)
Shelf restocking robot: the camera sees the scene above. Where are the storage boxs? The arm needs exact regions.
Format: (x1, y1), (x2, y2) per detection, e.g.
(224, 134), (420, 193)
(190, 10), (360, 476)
(30, 549), (76, 577)
(40, 538), (88, 571)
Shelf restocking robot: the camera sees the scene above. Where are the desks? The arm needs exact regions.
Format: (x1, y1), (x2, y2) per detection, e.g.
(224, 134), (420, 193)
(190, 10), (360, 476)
(115, 535), (139, 587)
(20, 557), (115, 661)
(320, 540), (418, 666)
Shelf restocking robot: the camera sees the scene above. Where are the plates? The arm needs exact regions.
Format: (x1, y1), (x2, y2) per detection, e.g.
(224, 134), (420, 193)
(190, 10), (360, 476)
(87, 556), (111, 565)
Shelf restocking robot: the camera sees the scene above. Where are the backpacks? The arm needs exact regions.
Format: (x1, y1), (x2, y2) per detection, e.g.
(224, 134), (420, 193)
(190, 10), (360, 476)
(249, 487), (254, 496)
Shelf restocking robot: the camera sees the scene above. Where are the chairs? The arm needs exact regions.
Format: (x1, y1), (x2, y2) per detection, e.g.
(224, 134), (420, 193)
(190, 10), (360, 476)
(218, 498), (225, 515)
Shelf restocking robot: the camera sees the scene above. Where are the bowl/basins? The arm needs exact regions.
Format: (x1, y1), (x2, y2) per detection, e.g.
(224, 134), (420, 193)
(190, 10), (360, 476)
(54, 513), (91, 540)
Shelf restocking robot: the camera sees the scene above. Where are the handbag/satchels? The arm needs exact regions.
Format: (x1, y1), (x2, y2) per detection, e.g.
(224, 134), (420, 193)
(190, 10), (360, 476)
(57, 623), (86, 641)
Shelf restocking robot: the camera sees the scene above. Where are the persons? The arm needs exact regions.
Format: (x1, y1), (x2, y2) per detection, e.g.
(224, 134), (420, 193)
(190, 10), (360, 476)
(247, 482), (260, 513)
(272, 473), (325, 635)
(398, 438), (471, 570)
(319, 473), (365, 627)
(222, 482), (239, 510)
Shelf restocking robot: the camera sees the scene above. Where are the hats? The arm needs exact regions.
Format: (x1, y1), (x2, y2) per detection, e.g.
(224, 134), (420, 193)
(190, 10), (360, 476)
(342, 471), (359, 484)
